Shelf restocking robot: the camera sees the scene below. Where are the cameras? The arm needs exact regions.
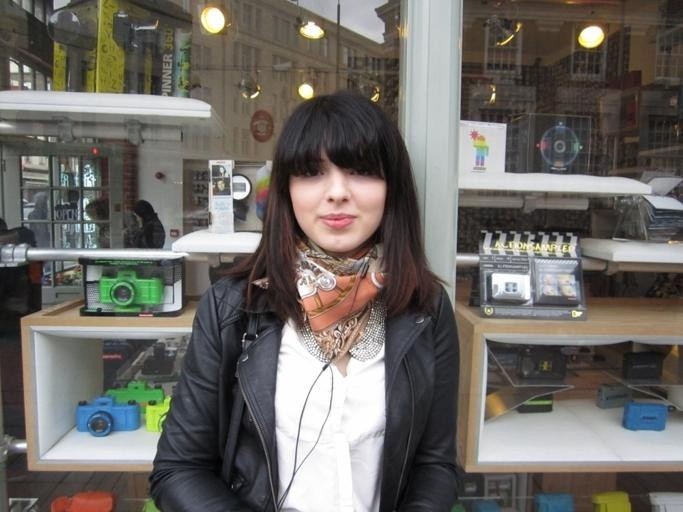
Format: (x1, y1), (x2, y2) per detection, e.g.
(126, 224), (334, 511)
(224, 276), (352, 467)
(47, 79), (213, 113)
(98, 268), (165, 313)
(104, 380), (165, 404)
(145, 394), (173, 433)
(74, 396), (142, 437)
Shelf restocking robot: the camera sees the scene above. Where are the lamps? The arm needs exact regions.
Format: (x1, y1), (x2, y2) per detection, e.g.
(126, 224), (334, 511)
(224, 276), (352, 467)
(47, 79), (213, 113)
(298, 79), (317, 99)
(470, 84), (497, 102)
(368, 86), (380, 103)
(200, 5), (231, 34)
(298, 5), (327, 38)
(493, 15), (523, 45)
(239, 74), (262, 100)
(578, 21), (605, 49)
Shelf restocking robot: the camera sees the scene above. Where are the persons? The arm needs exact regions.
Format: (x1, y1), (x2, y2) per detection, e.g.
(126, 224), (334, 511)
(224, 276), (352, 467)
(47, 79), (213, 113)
(218, 167), (229, 177)
(212, 180), (229, 195)
(87, 200), (132, 248)
(134, 199), (165, 248)
(145, 87), (463, 512)
(28, 192), (52, 274)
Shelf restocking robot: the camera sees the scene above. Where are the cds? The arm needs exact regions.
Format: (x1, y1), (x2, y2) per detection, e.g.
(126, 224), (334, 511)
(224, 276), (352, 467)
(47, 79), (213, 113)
(540, 124), (582, 168)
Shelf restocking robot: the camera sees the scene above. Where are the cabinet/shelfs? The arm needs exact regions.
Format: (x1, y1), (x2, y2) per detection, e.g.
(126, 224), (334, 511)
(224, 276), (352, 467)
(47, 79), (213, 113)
(21, 297), (203, 472)
(454, 296), (683, 473)
